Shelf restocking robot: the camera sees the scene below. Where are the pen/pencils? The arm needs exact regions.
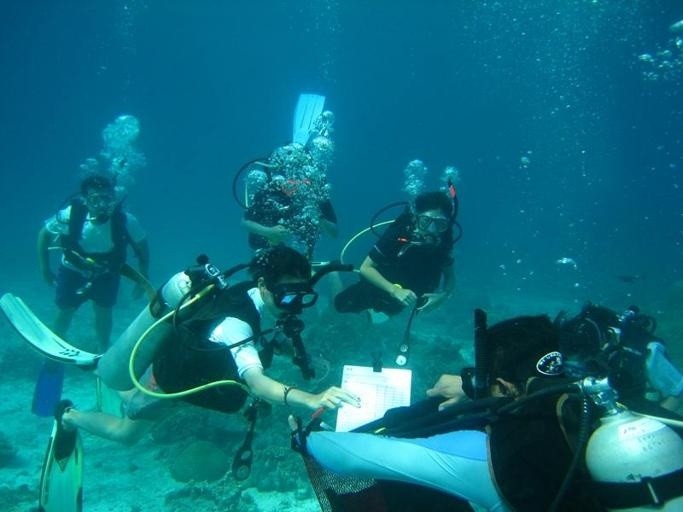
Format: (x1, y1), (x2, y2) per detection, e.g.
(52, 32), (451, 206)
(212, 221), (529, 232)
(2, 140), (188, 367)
(311, 407), (325, 418)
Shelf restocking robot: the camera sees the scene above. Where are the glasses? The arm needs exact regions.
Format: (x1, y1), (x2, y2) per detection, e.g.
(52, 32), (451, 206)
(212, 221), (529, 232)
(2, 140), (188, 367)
(415, 215), (450, 233)
(264, 279), (318, 308)
(87, 194), (116, 208)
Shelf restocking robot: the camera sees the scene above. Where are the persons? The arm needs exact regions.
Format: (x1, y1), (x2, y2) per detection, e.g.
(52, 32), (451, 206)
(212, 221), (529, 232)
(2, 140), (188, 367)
(333, 191), (458, 314)
(33, 174), (149, 417)
(239, 143), (339, 261)
(2, 246), (364, 511)
